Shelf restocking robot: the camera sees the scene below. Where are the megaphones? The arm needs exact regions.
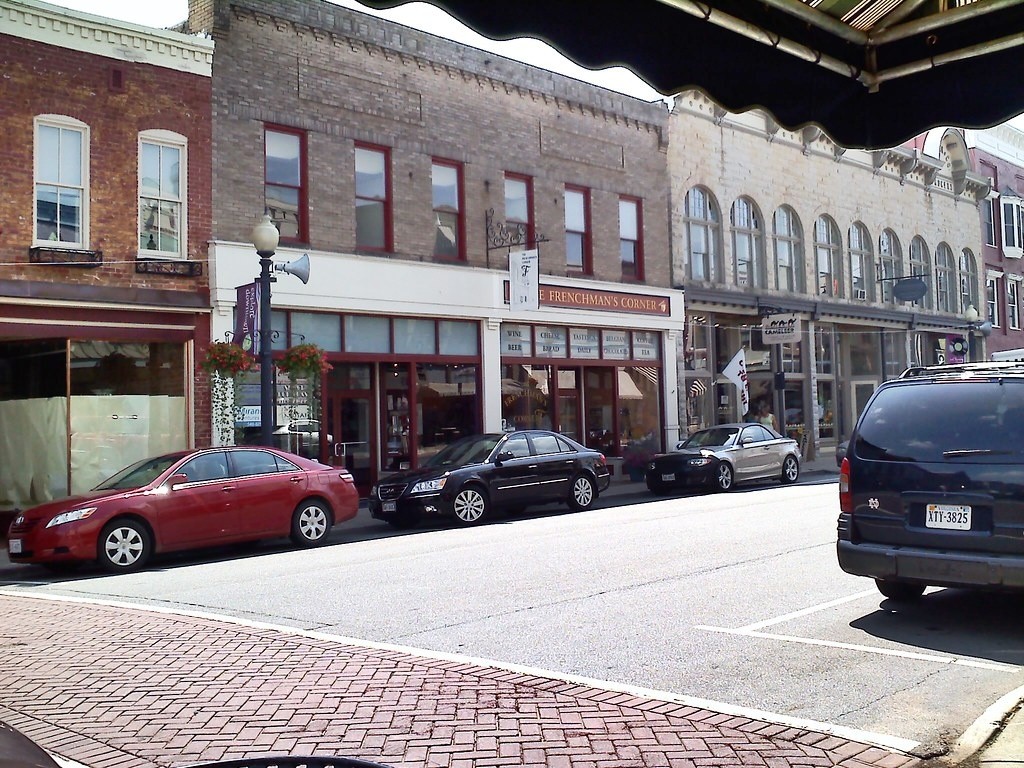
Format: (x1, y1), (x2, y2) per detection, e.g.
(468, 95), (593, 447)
(275, 252), (310, 284)
(973, 321), (993, 337)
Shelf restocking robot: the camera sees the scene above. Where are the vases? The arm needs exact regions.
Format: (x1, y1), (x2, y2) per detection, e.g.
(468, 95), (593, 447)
(217, 369), (233, 378)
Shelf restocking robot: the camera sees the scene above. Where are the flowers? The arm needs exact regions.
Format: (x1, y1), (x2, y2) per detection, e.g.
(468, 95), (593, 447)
(196, 341), (259, 372)
(271, 343), (334, 380)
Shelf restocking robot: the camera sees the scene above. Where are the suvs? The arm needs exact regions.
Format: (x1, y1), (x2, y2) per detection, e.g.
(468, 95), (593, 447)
(835, 346), (1023, 603)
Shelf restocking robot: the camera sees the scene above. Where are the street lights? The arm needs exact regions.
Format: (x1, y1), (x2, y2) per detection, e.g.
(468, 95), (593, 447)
(252, 208), (281, 451)
(965, 303), (981, 366)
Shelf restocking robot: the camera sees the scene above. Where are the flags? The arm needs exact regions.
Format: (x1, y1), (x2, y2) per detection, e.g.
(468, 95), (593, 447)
(720, 346), (751, 416)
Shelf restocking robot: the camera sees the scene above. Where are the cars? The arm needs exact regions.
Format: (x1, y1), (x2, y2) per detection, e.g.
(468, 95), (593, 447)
(275, 419), (335, 457)
(368, 428), (610, 529)
(645, 423), (802, 493)
(6, 446), (362, 576)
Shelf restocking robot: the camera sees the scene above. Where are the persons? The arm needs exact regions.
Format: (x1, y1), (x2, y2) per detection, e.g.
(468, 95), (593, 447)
(754, 401), (780, 434)
